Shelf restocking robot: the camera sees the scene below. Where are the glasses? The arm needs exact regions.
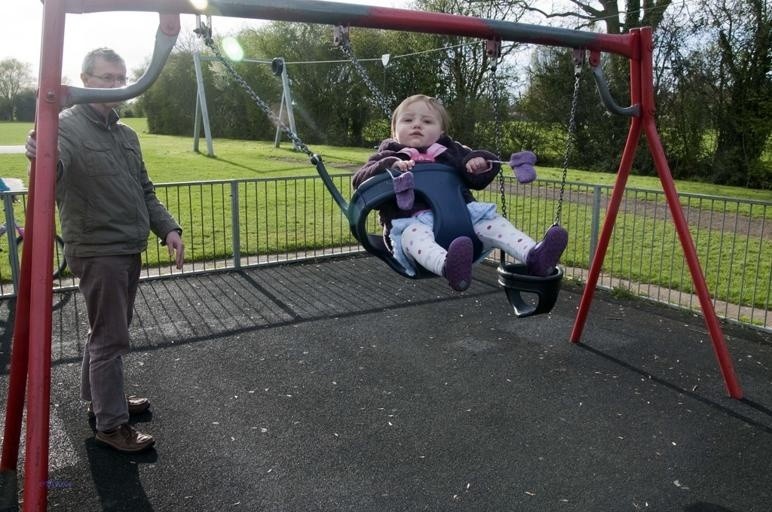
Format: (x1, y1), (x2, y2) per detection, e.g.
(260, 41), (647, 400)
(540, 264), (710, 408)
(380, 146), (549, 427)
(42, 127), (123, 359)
(85, 73), (128, 83)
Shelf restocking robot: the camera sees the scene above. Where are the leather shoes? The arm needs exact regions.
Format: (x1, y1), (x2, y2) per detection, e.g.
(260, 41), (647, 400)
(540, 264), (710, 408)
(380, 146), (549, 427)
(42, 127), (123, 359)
(96, 424), (154, 451)
(87, 395), (150, 415)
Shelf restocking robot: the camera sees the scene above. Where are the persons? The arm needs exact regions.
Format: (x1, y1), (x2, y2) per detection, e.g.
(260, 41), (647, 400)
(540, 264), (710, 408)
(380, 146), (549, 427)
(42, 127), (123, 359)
(24, 45), (186, 450)
(352, 93), (569, 294)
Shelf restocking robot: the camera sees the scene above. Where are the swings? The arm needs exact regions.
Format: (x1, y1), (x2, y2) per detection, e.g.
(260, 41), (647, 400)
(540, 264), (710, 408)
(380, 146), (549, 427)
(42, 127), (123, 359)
(196, 23), (495, 277)
(490, 56), (582, 317)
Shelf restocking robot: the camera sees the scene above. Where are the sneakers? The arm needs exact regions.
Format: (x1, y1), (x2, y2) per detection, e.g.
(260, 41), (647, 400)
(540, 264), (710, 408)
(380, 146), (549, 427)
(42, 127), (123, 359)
(527, 226), (568, 275)
(443, 236), (473, 291)
